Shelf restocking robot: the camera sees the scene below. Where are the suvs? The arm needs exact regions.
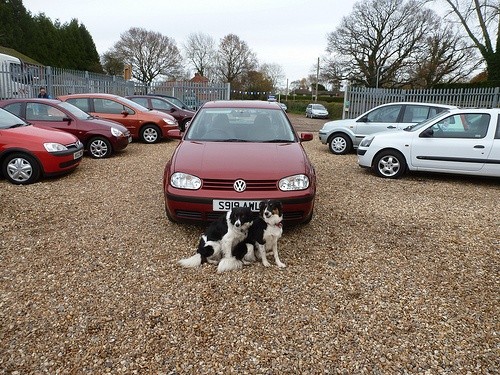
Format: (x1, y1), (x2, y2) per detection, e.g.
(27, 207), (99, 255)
(318, 101), (461, 155)
(357, 108), (500, 178)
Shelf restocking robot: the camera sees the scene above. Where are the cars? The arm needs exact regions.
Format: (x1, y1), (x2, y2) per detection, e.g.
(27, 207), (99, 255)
(0, 93), (197, 184)
(163, 100), (317, 226)
(304, 103), (330, 119)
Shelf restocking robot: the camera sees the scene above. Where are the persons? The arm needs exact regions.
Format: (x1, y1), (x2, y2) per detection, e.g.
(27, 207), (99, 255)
(140, 88), (145, 95)
(191, 91), (198, 111)
(38, 88), (48, 116)
(104, 70), (116, 94)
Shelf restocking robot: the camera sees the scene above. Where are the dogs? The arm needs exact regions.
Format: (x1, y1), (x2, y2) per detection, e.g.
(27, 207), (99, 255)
(180, 207), (254, 268)
(217, 200), (287, 274)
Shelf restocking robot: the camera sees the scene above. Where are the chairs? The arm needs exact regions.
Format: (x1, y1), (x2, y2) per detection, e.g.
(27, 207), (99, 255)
(248, 114), (273, 138)
(212, 113), (234, 138)
(38, 105), (48, 116)
